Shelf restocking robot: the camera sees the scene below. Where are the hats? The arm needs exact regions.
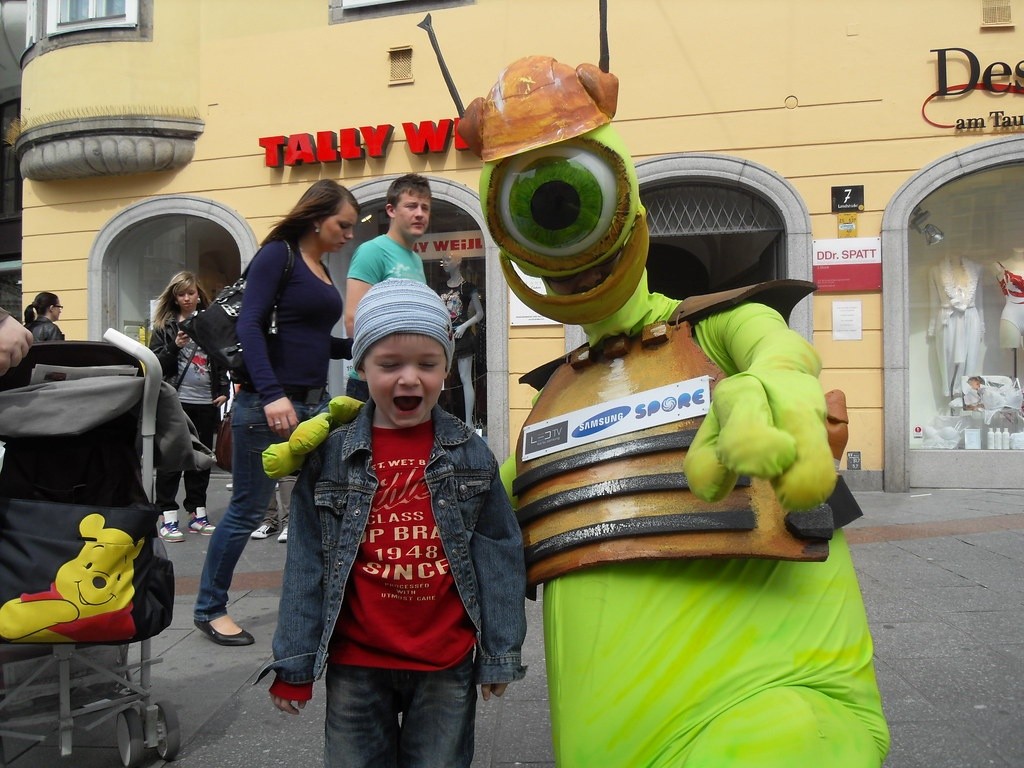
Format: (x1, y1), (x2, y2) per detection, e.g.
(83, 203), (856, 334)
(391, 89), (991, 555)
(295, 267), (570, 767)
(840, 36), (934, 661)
(352, 278), (455, 371)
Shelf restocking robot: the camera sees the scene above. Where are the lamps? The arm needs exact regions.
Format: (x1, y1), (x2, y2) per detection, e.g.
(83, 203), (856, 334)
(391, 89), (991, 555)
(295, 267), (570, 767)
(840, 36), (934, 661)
(910, 208), (944, 246)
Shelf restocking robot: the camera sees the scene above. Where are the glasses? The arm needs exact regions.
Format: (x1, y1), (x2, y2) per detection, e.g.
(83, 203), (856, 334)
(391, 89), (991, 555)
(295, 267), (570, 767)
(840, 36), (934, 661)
(54, 304), (63, 309)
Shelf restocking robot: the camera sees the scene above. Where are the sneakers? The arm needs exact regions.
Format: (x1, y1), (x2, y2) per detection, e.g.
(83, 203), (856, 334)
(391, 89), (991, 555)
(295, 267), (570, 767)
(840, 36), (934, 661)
(250, 524), (279, 538)
(278, 526), (287, 543)
(159, 519), (185, 543)
(188, 514), (216, 535)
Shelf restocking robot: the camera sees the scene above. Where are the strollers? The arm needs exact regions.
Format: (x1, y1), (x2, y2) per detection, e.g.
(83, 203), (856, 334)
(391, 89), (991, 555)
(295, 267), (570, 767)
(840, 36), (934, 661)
(0, 329), (179, 768)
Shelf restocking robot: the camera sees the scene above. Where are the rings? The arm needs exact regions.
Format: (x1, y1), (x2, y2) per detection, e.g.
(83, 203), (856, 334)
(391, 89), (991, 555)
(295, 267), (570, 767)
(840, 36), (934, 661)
(275, 421), (281, 425)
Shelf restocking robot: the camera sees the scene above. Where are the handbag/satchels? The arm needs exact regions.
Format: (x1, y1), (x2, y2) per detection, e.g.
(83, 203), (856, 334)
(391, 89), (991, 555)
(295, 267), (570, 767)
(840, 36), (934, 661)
(189, 236), (295, 384)
(215, 412), (233, 472)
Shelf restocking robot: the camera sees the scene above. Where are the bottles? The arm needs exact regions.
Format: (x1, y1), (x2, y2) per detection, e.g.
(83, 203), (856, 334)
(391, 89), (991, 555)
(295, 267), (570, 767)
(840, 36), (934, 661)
(987, 428), (994, 449)
(1002, 428), (1009, 449)
(994, 428), (1002, 449)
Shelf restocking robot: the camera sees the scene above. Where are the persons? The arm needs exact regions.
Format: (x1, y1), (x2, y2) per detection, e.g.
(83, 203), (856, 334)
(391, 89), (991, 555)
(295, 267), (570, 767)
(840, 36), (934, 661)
(928, 251), (987, 397)
(226, 383), (302, 541)
(261, 57), (890, 768)
(24, 292), (65, 343)
(0, 307), (33, 376)
(195, 178), (361, 647)
(435, 251), (483, 430)
(148, 270), (231, 543)
(991, 246), (1024, 348)
(344, 174), (431, 403)
(254, 279), (529, 768)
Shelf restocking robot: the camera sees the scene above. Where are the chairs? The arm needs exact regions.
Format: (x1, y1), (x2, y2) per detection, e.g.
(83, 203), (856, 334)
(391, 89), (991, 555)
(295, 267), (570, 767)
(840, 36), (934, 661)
(950, 375), (1024, 429)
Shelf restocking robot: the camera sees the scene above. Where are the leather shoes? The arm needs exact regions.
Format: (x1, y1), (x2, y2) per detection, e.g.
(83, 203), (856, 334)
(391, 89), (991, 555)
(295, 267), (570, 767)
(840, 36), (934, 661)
(193, 618), (255, 646)
